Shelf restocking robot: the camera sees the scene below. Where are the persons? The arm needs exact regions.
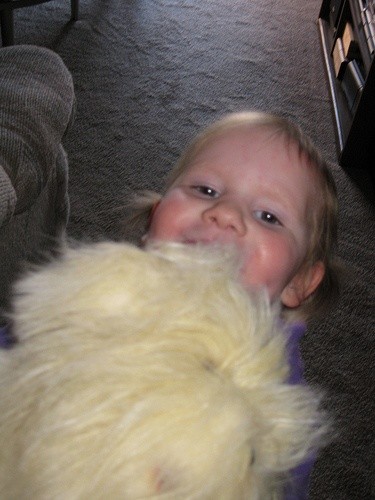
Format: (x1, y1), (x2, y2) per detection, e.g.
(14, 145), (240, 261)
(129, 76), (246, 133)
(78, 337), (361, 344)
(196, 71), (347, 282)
(4, 109), (342, 500)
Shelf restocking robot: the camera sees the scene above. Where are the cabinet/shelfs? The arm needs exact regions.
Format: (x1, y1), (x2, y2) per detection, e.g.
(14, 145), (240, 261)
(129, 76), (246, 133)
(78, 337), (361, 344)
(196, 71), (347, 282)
(318, 0), (375, 167)
